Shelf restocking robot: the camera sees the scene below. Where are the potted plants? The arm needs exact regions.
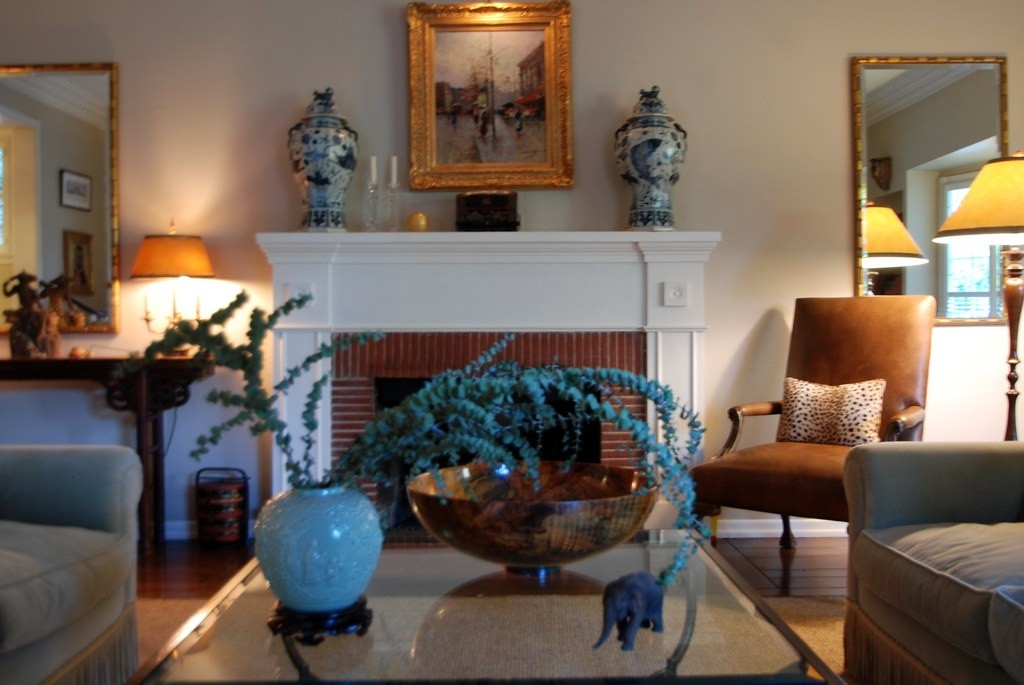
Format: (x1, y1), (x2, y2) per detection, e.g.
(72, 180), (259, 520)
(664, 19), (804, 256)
(142, 282), (711, 617)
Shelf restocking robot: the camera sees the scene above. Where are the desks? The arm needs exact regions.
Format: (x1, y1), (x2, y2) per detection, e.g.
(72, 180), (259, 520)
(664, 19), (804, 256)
(0, 352), (216, 568)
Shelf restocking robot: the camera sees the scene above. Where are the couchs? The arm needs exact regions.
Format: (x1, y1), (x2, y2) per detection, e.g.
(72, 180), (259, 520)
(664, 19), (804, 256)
(841, 440), (1024, 685)
(0, 443), (144, 685)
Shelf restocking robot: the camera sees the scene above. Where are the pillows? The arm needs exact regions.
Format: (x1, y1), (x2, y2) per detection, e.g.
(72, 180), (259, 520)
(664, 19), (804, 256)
(775, 377), (886, 447)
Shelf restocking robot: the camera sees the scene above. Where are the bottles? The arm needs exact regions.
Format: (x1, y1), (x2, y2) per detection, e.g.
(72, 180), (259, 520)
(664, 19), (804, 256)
(286, 86), (360, 233)
(613, 85), (688, 232)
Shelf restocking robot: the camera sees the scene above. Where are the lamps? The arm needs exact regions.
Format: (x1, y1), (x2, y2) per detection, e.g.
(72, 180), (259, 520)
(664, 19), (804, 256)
(128, 215), (217, 357)
(864, 199), (929, 295)
(932, 148), (1024, 442)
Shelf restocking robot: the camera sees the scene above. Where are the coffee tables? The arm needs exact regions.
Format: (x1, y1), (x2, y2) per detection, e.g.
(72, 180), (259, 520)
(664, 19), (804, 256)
(123, 527), (845, 685)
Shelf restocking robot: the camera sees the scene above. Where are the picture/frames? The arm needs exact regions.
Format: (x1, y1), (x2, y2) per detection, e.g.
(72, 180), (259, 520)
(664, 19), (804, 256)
(59, 167), (93, 213)
(62, 229), (96, 296)
(404, 0), (576, 194)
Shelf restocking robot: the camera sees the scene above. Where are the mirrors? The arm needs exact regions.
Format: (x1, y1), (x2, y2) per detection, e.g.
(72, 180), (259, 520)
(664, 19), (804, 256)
(0, 60), (121, 336)
(850, 55), (1012, 327)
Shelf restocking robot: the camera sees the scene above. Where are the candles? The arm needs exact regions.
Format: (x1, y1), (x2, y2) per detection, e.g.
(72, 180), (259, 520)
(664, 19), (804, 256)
(389, 154), (398, 186)
(370, 154), (379, 185)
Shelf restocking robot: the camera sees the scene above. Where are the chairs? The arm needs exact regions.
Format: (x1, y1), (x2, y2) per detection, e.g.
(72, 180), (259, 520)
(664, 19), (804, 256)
(689, 295), (937, 549)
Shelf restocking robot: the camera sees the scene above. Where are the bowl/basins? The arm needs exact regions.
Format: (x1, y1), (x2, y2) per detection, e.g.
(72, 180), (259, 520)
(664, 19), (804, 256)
(405, 460), (659, 580)
